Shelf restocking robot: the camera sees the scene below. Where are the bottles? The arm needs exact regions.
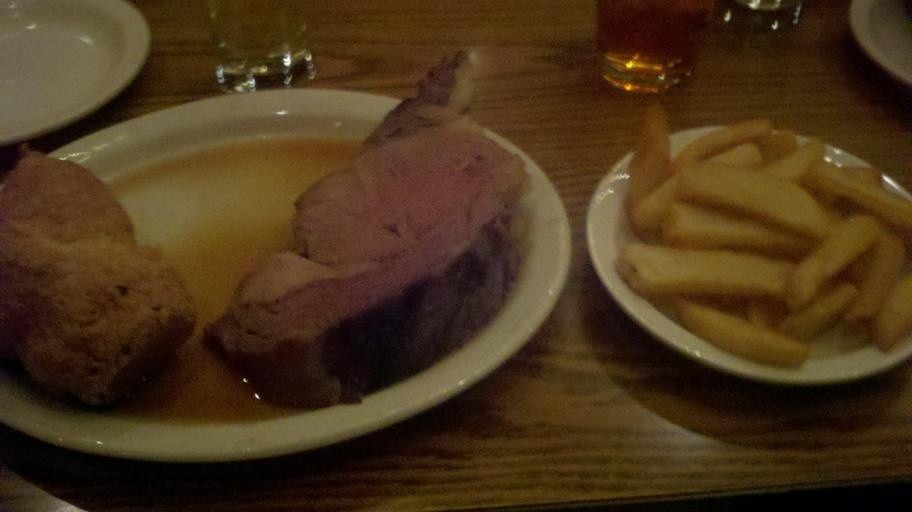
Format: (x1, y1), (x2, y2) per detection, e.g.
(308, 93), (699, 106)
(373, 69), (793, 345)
(714, 0), (804, 41)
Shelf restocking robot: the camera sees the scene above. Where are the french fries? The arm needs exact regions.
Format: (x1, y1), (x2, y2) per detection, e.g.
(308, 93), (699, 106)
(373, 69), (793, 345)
(619, 101), (911, 367)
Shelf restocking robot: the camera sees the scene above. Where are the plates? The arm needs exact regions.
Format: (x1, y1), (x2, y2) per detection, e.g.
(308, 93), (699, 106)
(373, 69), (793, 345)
(0, 1), (152, 145)
(1, 89), (572, 465)
(585, 124), (912, 386)
(849, 0), (912, 88)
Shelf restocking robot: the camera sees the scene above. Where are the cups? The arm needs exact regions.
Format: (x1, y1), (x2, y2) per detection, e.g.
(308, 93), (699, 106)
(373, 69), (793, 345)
(206, 0), (319, 95)
(595, 0), (701, 97)
